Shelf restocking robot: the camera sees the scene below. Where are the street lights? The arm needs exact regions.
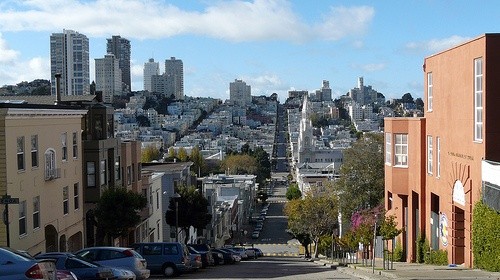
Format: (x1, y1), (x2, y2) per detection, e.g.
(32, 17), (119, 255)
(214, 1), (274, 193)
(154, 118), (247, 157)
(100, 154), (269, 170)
(170, 193), (181, 242)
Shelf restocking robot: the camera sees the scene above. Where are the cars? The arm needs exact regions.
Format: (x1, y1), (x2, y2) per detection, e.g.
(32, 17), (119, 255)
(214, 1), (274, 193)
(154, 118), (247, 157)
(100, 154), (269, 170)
(1, 199), (274, 280)
